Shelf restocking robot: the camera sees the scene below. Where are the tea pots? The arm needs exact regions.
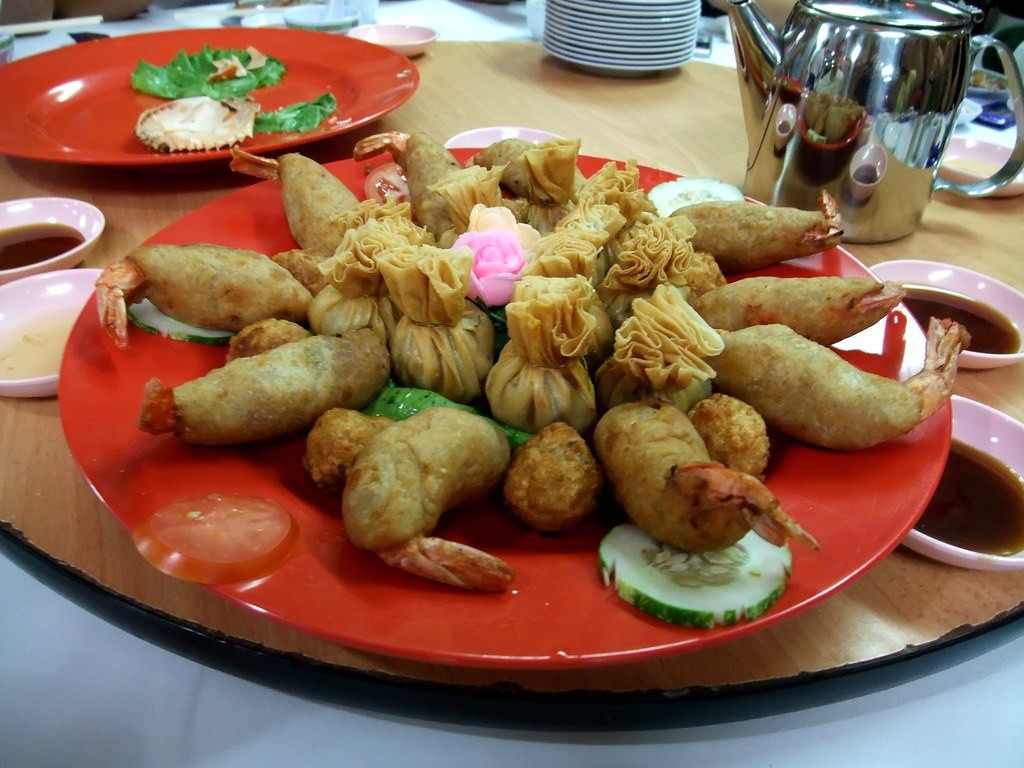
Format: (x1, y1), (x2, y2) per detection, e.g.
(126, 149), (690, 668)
(728, 0), (1024, 246)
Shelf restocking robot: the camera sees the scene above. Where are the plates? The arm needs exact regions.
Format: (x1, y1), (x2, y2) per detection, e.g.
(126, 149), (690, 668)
(773, 103), (796, 152)
(779, 77), (803, 100)
(544, 0), (702, 75)
(0, 197), (106, 284)
(282, 4), (360, 36)
(56, 148), (953, 669)
(243, 13), (350, 36)
(938, 137), (1024, 199)
(849, 143), (887, 201)
(443, 126), (580, 152)
(797, 96), (867, 152)
(0, 267), (124, 398)
(903, 394), (1024, 574)
(348, 24), (436, 57)
(867, 258), (1024, 370)
(0, 28), (421, 168)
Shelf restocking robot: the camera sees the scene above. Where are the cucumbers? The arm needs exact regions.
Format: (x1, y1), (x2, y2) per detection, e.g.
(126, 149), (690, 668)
(127, 298), (237, 345)
(597, 521), (791, 629)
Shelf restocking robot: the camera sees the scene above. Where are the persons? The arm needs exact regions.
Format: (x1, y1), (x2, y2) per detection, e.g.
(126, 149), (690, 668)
(805, 38), (830, 91)
(815, 39), (846, 94)
(899, 66), (917, 112)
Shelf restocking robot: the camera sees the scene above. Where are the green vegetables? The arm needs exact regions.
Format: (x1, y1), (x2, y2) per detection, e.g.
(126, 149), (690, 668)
(129, 46), (337, 135)
(344, 296), (532, 447)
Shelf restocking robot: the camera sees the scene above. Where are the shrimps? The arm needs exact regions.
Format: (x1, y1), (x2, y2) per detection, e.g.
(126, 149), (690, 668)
(93, 129), (973, 592)
